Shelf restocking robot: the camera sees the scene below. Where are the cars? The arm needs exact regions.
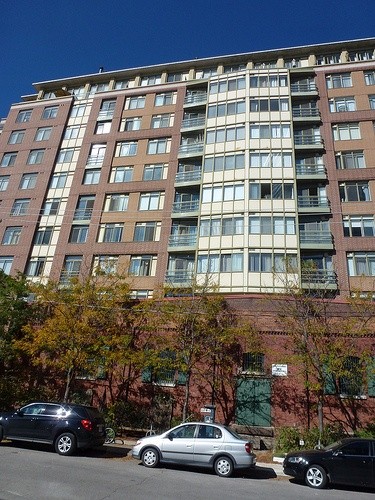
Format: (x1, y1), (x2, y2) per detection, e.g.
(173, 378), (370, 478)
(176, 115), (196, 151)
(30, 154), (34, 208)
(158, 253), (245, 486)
(284, 436), (375, 490)
(131, 421), (258, 477)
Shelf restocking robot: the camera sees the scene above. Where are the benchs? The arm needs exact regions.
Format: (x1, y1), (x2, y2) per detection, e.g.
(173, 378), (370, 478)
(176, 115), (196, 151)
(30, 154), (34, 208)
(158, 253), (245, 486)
(117, 426), (157, 438)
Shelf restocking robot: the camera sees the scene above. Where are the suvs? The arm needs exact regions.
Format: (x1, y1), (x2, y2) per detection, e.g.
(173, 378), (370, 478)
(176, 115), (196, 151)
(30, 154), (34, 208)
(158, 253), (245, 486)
(0, 400), (106, 456)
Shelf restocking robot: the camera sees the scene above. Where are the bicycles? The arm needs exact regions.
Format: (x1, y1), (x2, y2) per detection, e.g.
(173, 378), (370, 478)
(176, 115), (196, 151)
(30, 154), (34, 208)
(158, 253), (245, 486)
(105, 427), (116, 443)
